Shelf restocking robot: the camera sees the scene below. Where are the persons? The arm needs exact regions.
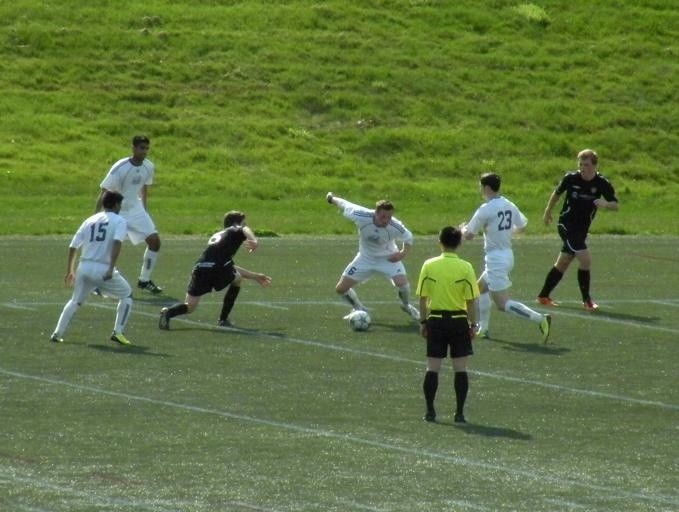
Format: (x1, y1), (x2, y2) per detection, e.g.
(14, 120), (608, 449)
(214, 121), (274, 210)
(536, 148), (618, 312)
(416, 226), (479, 424)
(326, 192), (421, 321)
(88, 135), (163, 294)
(48, 190), (133, 345)
(159, 210), (272, 330)
(460, 171), (551, 346)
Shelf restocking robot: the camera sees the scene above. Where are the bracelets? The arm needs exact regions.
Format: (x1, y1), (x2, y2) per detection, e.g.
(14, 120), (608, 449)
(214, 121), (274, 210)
(470, 323), (479, 328)
(420, 319), (427, 324)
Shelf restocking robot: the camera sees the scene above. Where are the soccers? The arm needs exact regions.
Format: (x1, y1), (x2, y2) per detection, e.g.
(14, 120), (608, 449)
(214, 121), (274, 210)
(348, 310), (371, 332)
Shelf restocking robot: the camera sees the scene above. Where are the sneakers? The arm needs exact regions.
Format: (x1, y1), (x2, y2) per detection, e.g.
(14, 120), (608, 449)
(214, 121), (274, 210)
(344, 306), (370, 319)
(51, 332), (64, 343)
(137, 279), (162, 293)
(159, 307), (171, 330)
(110, 330), (131, 345)
(400, 302), (422, 321)
(216, 319), (233, 327)
(422, 413), (436, 424)
(582, 297), (599, 312)
(454, 411), (470, 426)
(475, 328), (490, 339)
(535, 295), (560, 308)
(539, 314), (552, 346)
